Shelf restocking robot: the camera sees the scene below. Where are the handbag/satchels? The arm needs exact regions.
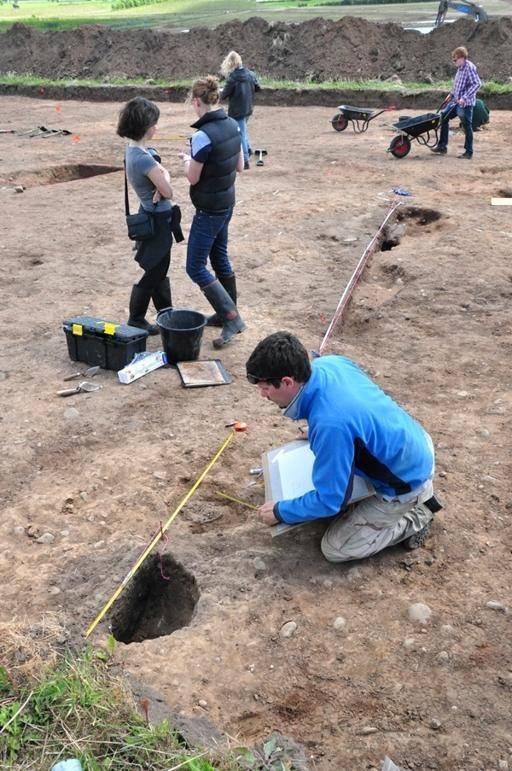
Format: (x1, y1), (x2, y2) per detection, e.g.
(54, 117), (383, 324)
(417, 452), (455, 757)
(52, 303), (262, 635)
(126, 211), (156, 241)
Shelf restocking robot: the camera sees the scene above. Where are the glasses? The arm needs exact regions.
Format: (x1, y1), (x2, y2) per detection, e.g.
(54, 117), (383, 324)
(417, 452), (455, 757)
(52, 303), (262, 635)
(453, 57), (460, 63)
(246, 373), (291, 384)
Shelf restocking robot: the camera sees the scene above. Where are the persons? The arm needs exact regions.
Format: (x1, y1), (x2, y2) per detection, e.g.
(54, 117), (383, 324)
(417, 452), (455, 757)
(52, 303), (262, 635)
(115, 97), (185, 336)
(178, 74), (250, 348)
(217, 49), (259, 170)
(248, 332), (445, 567)
(430, 47), (481, 159)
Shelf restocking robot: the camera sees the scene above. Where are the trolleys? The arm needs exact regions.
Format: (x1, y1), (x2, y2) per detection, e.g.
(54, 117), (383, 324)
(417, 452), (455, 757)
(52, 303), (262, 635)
(327, 103), (400, 135)
(386, 94), (461, 159)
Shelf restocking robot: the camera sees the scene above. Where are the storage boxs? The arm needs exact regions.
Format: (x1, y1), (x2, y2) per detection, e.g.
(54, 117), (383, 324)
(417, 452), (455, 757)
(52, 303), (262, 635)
(63, 316), (149, 372)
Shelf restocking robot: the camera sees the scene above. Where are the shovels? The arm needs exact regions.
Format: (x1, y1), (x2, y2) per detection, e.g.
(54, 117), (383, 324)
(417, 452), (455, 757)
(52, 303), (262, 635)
(17, 126), (48, 136)
(56, 381), (103, 397)
(63, 366), (100, 381)
(30, 130), (58, 137)
(42, 129), (72, 138)
(254, 149), (267, 166)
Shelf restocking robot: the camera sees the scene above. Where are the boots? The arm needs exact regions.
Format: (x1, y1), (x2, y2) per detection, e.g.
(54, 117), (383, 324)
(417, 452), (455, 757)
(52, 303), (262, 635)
(200, 277), (247, 349)
(152, 277), (172, 312)
(127, 284), (160, 335)
(204, 272), (237, 326)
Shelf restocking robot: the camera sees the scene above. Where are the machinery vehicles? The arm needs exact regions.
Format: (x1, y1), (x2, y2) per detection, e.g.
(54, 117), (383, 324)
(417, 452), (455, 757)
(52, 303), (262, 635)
(434, 0), (489, 25)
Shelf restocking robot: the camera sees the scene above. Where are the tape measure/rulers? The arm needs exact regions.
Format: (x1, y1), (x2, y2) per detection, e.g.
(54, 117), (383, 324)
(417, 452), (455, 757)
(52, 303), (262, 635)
(81, 420), (248, 640)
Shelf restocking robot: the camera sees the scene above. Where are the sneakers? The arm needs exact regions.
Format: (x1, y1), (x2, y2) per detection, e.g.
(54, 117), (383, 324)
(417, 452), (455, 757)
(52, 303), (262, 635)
(401, 518), (434, 549)
(423, 495), (443, 513)
(455, 150), (472, 159)
(430, 145), (447, 154)
(248, 148), (253, 156)
(244, 161), (249, 169)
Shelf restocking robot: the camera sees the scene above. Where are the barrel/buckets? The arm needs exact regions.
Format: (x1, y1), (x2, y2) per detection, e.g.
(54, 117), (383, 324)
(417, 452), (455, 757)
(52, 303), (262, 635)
(153, 306), (208, 365)
